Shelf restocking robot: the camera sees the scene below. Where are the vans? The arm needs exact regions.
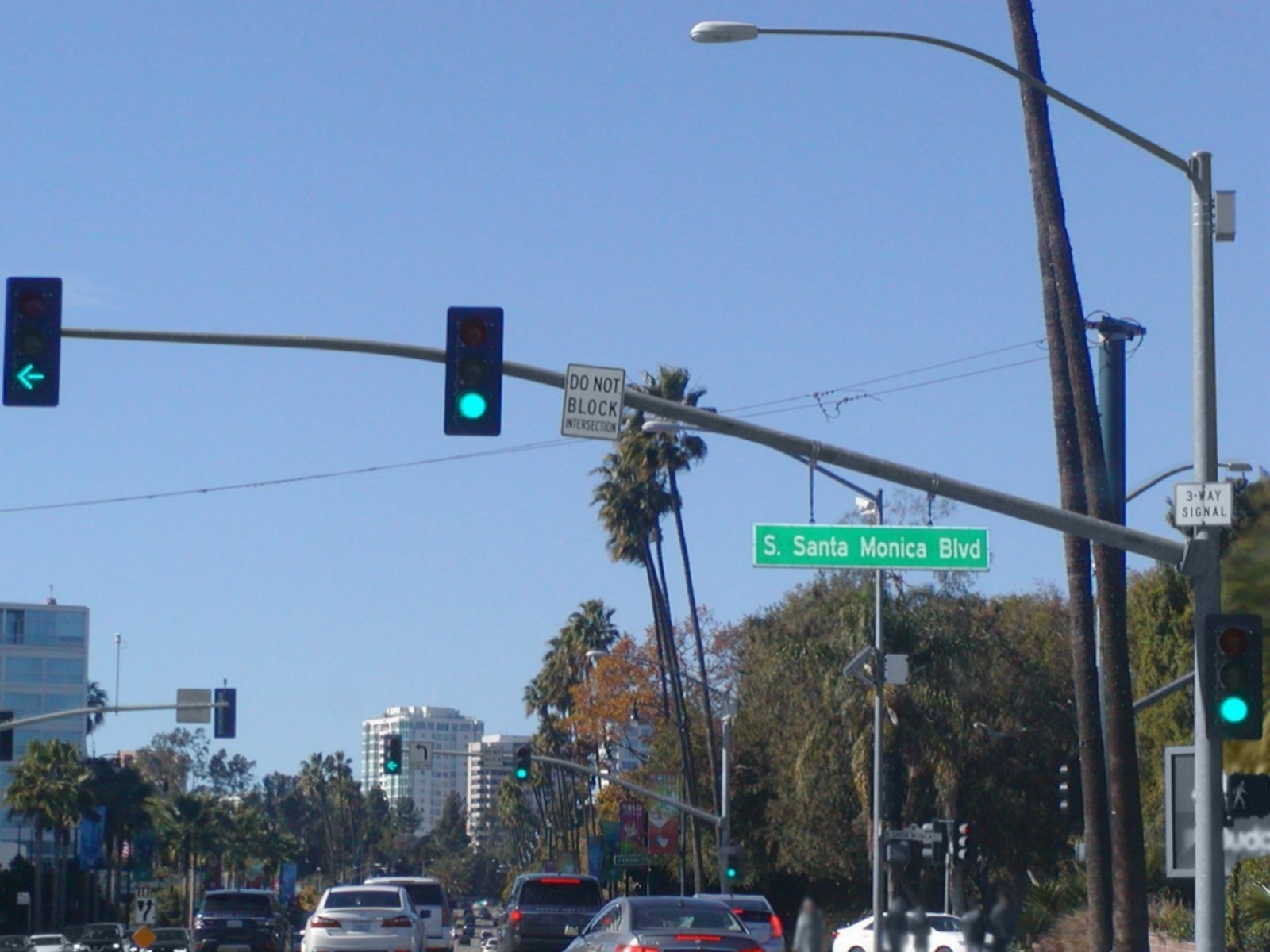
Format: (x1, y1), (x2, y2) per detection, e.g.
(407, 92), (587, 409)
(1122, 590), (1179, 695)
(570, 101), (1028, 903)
(364, 876), (457, 952)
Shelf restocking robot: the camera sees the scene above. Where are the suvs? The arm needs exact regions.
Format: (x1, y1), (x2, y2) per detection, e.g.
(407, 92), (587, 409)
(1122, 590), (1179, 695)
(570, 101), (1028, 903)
(497, 873), (610, 952)
(74, 922), (124, 952)
(150, 927), (191, 952)
(193, 887), (289, 952)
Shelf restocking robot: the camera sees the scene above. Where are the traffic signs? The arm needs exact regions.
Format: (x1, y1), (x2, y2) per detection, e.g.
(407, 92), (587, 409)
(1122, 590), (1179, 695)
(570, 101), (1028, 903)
(408, 741), (433, 772)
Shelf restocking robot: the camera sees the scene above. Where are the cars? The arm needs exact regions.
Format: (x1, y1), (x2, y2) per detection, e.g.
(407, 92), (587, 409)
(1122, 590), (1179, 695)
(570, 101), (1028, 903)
(0, 932), (94, 952)
(479, 928), (497, 952)
(299, 886), (432, 952)
(558, 893), (788, 952)
(453, 899), (488, 945)
(831, 912), (995, 952)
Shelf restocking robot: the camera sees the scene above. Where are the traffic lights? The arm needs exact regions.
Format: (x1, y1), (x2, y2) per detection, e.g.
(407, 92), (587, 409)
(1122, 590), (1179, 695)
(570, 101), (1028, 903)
(1057, 763), (1084, 835)
(512, 741), (533, 782)
(726, 853), (744, 885)
(444, 307), (504, 437)
(382, 735), (402, 774)
(2, 275), (62, 409)
(922, 820), (943, 862)
(1205, 613), (1263, 739)
(955, 818), (978, 865)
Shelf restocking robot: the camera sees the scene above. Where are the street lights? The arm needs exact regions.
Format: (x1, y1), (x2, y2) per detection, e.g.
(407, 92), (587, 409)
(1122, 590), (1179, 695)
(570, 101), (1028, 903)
(585, 649), (736, 896)
(689, 16), (1234, 952)
(639, 419), (886, 951)
